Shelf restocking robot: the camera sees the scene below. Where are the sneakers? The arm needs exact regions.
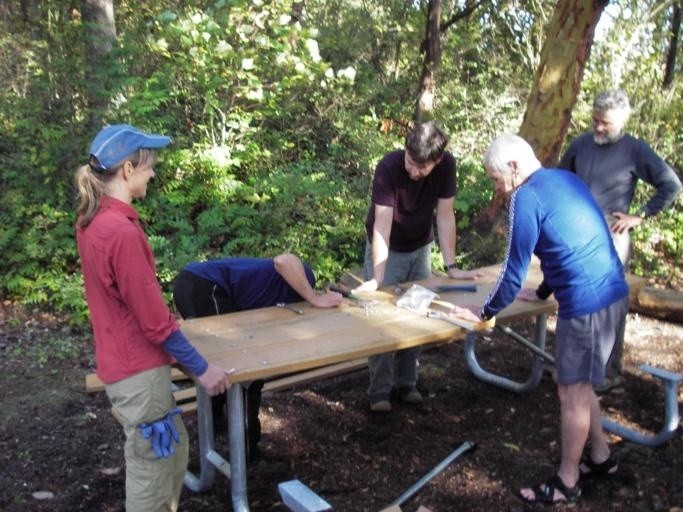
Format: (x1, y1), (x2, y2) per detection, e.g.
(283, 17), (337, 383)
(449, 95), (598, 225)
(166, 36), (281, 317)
(369, 393), (390, 411)
(401, 386), (423, 401)
(246, 459), (291, 480)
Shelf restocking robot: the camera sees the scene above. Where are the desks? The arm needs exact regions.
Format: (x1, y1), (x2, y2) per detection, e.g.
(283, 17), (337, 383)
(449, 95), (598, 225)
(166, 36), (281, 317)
(178, 253), (648, 512)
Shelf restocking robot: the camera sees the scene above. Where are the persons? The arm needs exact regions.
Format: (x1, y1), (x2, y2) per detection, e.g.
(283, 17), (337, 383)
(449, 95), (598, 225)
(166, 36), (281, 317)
(74, 123), (235, 512)
(351, 122), (485, 412)
(173, 253), (342, 469)
(557, 89), (683, 265)
(449, 133), (629, 506)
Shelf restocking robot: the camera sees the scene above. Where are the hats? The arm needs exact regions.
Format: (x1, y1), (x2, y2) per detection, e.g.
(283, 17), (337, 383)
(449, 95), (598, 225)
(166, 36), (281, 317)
(87, 125), (171, 170)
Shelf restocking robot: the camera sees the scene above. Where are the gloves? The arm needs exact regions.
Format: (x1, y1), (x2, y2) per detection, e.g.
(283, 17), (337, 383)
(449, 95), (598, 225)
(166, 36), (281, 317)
(141, 408), (182, 460)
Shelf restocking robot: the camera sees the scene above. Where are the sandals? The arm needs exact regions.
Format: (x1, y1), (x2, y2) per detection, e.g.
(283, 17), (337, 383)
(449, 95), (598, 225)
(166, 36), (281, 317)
(519, 473), (581, 504)
(580, 451), (621, 481)
